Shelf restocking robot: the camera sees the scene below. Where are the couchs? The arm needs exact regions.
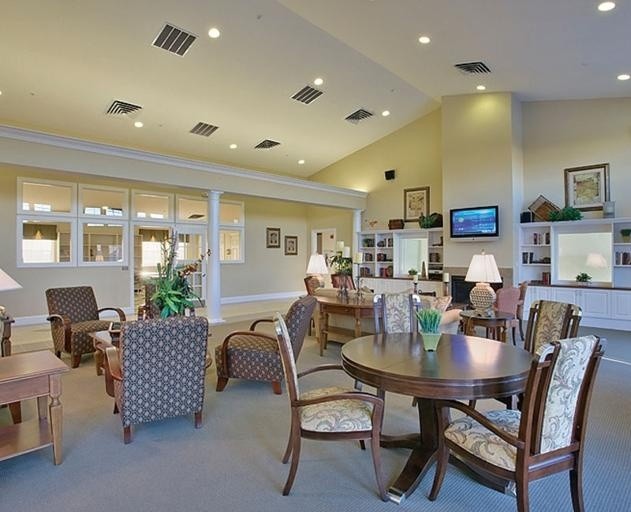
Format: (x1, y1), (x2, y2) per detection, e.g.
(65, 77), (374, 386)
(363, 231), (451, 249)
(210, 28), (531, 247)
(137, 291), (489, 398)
(315, 287), (464, 345)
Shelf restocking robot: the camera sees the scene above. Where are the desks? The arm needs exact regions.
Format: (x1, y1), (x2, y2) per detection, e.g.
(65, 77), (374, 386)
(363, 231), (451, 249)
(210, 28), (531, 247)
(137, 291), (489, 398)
(313, 296), (423, 356)
(447, 303), (469, 310)
(0, 348), (69, 465)
(459, 309), (515, 343)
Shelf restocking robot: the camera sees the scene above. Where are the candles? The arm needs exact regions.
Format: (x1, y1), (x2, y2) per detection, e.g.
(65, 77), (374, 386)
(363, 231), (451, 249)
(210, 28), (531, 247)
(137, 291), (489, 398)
(413, 275), (419, 283)
(336, 240), (344, 251)
(443, 273), (449, 282)
(355, 252), (363, 263)
(342, 247), (351, 258)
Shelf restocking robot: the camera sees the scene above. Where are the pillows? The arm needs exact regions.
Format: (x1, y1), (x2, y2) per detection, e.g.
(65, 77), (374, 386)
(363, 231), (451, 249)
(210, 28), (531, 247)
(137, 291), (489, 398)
(436, 296), (453, 313)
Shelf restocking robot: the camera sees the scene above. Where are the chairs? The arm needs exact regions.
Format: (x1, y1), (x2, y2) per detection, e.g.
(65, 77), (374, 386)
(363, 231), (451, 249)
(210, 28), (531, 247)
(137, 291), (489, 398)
(373, 291), (418, 407)
(305, 275), (321, 337)
(106, 316), (212, 444)
(466, 300), (582, 418)
(273, 311), (390, 504)
(331, 274), (374, 293)
(494, 281), (527, 346)
(45, 286), (126, 370)
(138, 283), (182, 321)
(428, 335), (607, 512)
(214, 295), (316, 395)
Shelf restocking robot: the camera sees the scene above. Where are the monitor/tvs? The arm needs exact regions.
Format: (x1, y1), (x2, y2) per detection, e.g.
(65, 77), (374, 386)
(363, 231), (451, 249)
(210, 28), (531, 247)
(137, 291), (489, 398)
(449, 205), (499, 242)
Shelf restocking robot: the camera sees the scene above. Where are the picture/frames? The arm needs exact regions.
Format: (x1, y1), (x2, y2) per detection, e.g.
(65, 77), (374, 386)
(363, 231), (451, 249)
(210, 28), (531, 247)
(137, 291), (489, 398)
(404, 186), (431, 222)
(563, 163), (610, 211)
(285, 235), (299, 255)
(265, 227), (281, 248)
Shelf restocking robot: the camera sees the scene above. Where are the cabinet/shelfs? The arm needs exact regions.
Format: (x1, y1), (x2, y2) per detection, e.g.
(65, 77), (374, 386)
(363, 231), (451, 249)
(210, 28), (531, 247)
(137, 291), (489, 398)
(519, 285), (577, 320)
(356, 227), (443, 282)
(578, 290), (631, 332)
(359, 278), (410, 295)
(515, 219), (631, 289)
(57, 224), (143, 271)
(410, 280), (446, 297)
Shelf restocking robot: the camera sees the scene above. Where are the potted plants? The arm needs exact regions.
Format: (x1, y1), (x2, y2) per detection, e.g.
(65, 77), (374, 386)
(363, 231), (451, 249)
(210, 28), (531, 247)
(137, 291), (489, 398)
(408, 268), (417, 279)
(413, 308), (443, 351)
(575, 272), (592, 287)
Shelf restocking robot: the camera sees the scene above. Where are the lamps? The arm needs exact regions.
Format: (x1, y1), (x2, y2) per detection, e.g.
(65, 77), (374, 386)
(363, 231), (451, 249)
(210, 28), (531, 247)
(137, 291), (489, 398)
(306, 252), (329, 289)
(0, 266), (23, 322)
(464, 249), (503, 318)
